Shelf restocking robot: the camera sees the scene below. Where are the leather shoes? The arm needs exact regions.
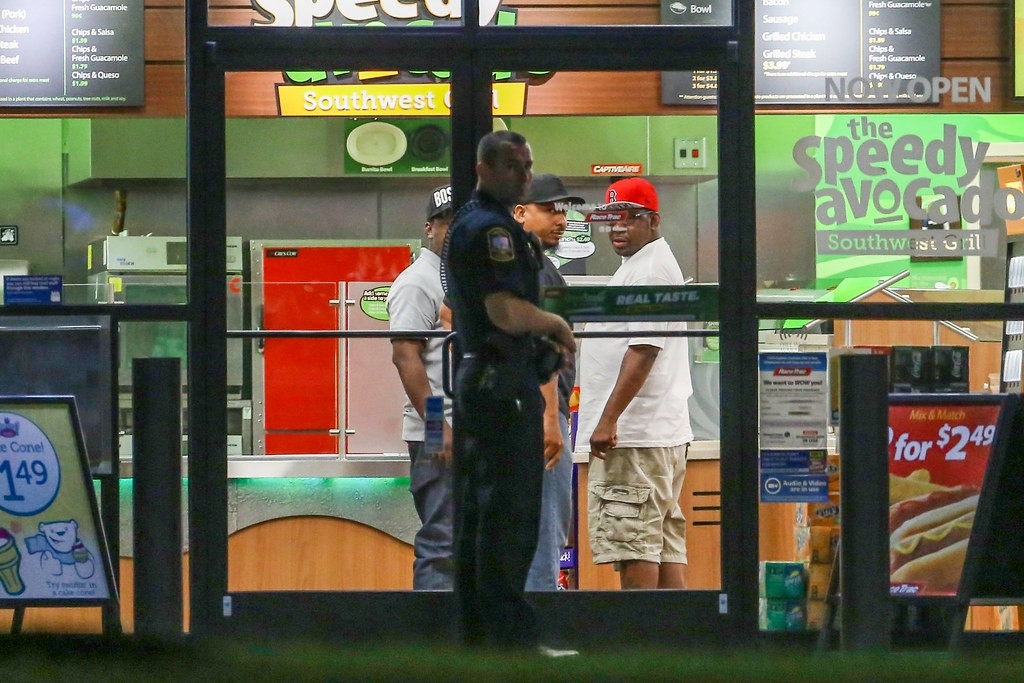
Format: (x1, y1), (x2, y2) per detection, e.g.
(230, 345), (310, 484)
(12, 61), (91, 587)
(497, 647), (579, 658)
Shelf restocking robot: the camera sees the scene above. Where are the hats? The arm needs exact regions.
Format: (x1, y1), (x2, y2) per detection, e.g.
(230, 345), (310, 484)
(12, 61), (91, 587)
(520, 173), (586, 204)
(596, 178), (658, 214)
(425, 185), (453, 221)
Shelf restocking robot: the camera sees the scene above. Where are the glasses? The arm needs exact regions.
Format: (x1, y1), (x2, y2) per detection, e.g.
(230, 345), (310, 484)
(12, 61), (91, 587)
(604, 211), (655, 218)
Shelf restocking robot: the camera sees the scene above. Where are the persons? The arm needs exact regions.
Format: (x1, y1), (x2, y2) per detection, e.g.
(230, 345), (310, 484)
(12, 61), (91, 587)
(573, 178), (694, 589)
(385, 129), (577, 592)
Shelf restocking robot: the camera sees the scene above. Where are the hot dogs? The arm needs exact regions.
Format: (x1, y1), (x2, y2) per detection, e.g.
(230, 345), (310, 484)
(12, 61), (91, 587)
(885, 483), (985, 590)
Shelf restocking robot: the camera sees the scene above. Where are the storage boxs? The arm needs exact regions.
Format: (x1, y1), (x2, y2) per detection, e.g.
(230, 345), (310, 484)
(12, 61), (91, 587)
(826, 455), (840, 490)
(855, 346), (971, 393)
(806, 601), (826, 631)
(760, 561), (804, 598)
(810, 527), (839, 564)
(809, 566), (834, 600)
(807, 492), (840, 527)
(758, 598), (806, 632)
